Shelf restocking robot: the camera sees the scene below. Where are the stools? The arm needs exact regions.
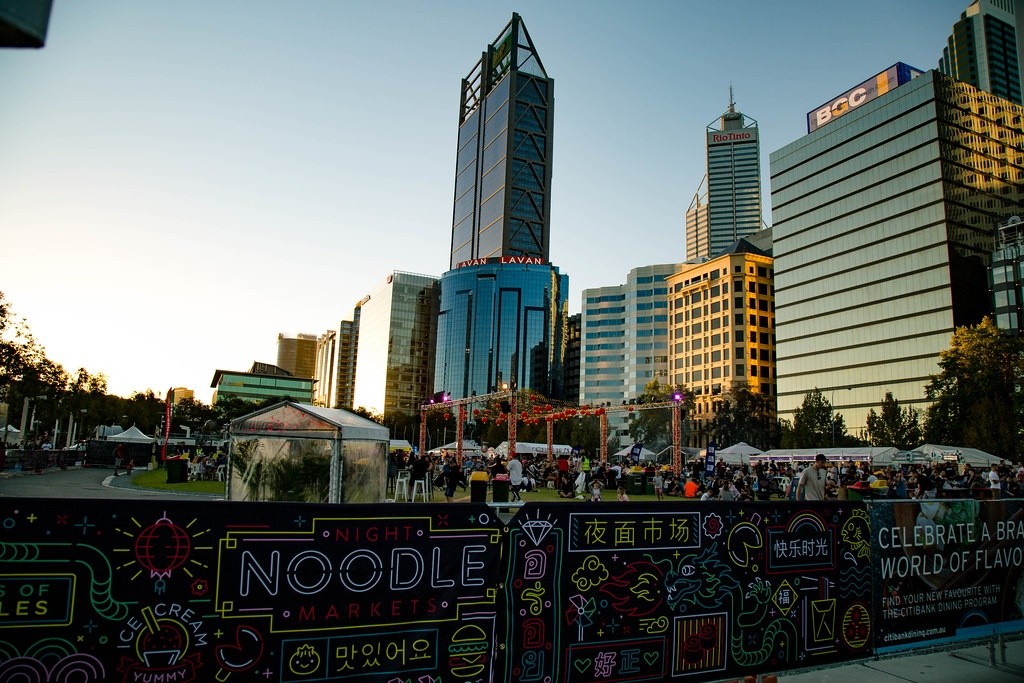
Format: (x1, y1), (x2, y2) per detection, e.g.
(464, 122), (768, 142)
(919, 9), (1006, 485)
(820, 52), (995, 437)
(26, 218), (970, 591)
(412, 480), (426, 503)
(395, 479), (409, 503)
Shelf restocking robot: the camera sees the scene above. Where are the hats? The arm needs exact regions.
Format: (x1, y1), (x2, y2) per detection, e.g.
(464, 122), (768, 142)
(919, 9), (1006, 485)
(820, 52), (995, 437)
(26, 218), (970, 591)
(579, 449), (585, 453)
(940, 471), (945, 475)
(573, 450), (579, 454)
(719, 457), (723, 459)
(848, 460), (854, 463)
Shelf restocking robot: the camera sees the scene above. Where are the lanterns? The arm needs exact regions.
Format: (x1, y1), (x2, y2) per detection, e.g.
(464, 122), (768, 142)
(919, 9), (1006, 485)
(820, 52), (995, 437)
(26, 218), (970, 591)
(444, 393), (634, 426)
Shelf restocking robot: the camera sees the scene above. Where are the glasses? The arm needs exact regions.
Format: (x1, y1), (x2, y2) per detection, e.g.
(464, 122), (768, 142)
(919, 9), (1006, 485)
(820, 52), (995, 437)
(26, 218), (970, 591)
(817, 472), (821, 480)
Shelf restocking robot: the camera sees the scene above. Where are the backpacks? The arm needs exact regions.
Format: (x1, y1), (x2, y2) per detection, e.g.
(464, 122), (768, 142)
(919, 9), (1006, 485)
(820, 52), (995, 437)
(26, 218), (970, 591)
(112, 448), (120, 458)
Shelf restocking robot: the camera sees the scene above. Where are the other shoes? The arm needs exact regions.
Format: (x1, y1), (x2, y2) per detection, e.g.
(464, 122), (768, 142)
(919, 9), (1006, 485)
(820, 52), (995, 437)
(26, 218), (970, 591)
(658, 498), (660, 501)
(519, 499), (523, 503)
(407, 498), (412, 502)
(464, 485), (466, 491)
(115, 472), (119, 476)
(114, 474), (116, 476)
(523, 489), (526, 492)
(519, 489), (523, 492)
(661, 496), (664, 499)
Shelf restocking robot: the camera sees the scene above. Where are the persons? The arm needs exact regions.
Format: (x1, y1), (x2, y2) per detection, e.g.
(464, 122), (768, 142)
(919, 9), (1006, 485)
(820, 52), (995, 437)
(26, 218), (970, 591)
(0, 432), (90, 470)
(109, 446), (1024, 505)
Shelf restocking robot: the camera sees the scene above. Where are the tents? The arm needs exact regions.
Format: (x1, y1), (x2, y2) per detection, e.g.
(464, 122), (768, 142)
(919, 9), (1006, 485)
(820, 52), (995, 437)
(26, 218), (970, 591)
(106, 422), (156, 443)
(1, 425), (20, 432)
(389, 438), (1015, 468)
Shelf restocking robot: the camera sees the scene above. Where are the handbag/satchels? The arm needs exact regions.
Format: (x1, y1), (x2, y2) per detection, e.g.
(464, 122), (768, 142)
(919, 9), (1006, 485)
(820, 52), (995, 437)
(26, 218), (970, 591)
(433, 475), (445, 487)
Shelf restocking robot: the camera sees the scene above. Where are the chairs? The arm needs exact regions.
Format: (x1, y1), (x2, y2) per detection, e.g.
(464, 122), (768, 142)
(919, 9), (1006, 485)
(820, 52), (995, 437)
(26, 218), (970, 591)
(216, 465), (225, 482)
(188, 463), (203, 482)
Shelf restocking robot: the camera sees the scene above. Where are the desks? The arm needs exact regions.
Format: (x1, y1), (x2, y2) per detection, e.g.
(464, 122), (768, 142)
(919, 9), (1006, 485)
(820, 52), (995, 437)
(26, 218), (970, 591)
(166, 459), (189, 484)
